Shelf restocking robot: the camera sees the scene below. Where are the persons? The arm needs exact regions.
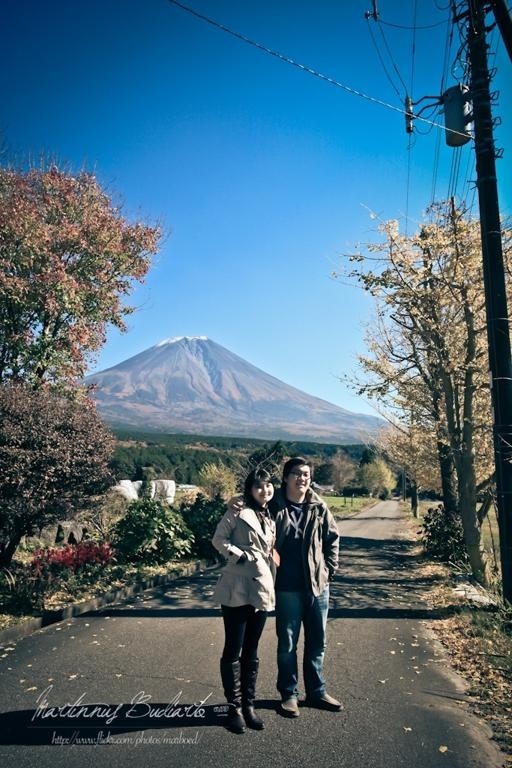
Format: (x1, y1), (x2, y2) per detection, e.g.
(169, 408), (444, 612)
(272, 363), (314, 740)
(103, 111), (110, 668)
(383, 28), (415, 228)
(212, 467), (281, 734)
(226, 457), (344, 717)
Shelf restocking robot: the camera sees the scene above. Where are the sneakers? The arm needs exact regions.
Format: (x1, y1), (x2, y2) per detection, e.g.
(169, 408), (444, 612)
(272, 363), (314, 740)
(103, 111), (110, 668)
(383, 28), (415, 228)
(306, 694), (344, 711)
(280, 699), (301, 718)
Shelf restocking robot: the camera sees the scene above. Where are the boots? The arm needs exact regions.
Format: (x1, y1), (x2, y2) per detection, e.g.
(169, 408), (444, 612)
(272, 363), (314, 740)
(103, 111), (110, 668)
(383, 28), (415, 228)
(220, 658), (246, 733)
(240, 658), (266, 730)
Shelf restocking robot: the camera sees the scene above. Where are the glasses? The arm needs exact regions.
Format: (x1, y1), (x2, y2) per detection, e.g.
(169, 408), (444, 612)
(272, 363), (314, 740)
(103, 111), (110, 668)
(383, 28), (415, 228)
(289, 472), (312, 480)
